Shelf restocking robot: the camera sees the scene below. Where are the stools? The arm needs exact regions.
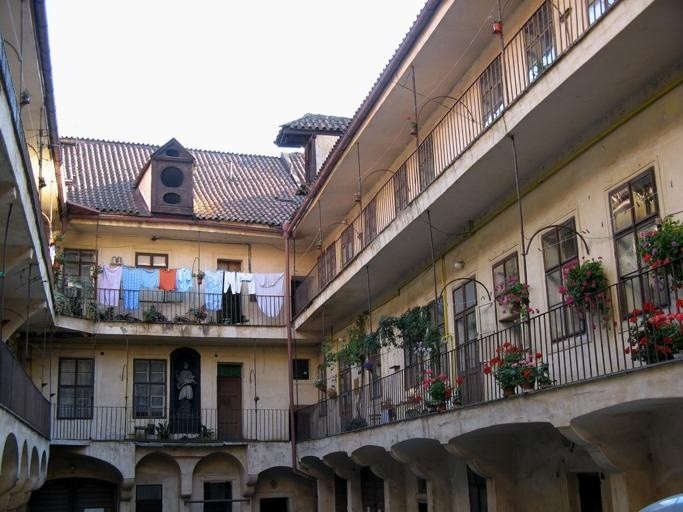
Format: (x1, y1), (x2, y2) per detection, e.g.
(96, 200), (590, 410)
(368, 414), (381, 426)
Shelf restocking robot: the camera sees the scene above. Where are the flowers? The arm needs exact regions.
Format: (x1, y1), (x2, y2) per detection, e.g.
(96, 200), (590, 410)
(623, 299), (683, 363)
(559, 254), (619, 334)
(636, 213), (683, 294)
(495, 274), (541, 318)
(481, 343), (550, 386)
(415, 369), (463, 402)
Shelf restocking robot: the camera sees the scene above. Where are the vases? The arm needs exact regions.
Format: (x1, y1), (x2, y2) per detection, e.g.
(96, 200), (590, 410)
(427, 403), (446, 413)
(503, 385), (516, 397)
(522, 380), (536, 390)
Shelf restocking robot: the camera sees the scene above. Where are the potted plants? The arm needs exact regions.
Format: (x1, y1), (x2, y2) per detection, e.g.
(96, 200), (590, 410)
(156, 422), (170, 441)
(200, 424), (214, 441)
(21, 89), (31, 104)
(53, 251), (65, 269)
(48, 230), (64, 246)
(197, 271), (205, 284)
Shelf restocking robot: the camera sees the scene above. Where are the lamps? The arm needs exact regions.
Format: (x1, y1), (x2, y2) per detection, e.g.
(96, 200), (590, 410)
(455, 260), (465, 269)
